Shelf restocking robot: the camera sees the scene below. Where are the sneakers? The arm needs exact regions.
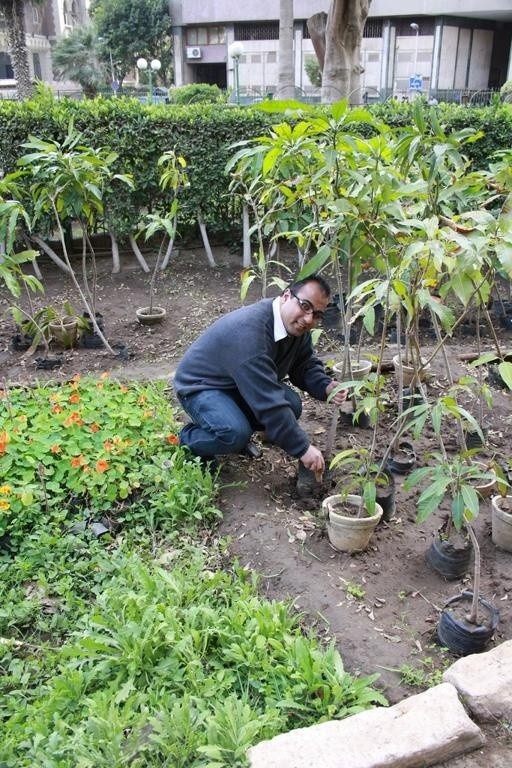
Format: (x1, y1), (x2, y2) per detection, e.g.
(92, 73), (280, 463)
(241, 441), (265, 459)
(194, 454), (220, 475)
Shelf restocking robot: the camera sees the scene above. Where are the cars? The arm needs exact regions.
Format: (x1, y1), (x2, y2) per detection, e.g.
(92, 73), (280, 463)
(1, 78), (19, 100)
(117, 85), (168, 96)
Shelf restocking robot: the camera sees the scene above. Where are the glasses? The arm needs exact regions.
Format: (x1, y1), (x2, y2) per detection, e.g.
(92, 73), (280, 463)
(291, 292), (326, 324)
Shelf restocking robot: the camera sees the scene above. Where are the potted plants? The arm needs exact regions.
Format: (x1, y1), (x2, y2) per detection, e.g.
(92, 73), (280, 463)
(225, 89), (512, 655)
(0, 117), (188, 348)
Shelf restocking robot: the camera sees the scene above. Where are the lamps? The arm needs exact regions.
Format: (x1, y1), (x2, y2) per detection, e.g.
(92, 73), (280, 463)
(186, 47), (203, 59)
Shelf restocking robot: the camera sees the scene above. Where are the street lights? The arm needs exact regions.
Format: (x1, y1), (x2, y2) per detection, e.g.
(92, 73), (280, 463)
(228, 41), (243, 107)
(405, 22), (421, 75)
(136, 56), (160, 104)
(98, 36), (121, 97)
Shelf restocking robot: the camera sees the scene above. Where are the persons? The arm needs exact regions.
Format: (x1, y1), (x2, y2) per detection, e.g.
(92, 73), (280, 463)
(171, 273), (350, 474)
